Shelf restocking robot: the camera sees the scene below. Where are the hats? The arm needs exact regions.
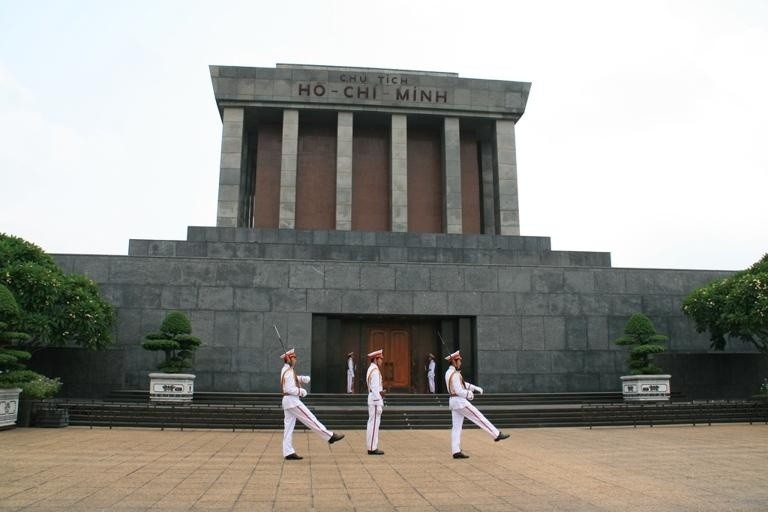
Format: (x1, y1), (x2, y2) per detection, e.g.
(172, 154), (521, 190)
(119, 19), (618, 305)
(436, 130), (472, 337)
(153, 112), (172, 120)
(279, 349), (462, 361)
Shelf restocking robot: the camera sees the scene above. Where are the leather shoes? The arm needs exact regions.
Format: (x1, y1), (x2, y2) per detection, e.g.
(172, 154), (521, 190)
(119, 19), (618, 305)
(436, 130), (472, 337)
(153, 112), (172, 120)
(285, 453), (302, 459)
(367, 449), (384, 454)
(494, 432), (509, 441)
(328, 433), (344, 444)
(453, 453), (468, 458)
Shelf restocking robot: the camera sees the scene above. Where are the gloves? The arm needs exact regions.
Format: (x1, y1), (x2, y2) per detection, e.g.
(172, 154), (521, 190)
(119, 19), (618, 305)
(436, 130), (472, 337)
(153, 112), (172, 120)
(376, 406), (382, 415)
(474, 387), (483, 395)
(466, 391), (473, 400)
(301, 389), (306, 397)
(301, 376), (310, 384)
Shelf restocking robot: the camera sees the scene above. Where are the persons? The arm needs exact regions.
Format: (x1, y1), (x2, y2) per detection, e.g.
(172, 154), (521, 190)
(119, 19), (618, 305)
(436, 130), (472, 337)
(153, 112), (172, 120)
(365, 349), (386, 455)
(427, 352), (436, 393)
(347, 351), (356, 393)
(444, 350), (510, 459)
(280, 348), (345, 460)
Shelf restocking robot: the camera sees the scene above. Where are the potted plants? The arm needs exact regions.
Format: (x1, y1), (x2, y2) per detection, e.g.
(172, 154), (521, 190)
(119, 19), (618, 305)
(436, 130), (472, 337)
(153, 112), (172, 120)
(614, 312), (672, 402)
(141, 311), (202, 403)
(0, 322), (64, 431)
